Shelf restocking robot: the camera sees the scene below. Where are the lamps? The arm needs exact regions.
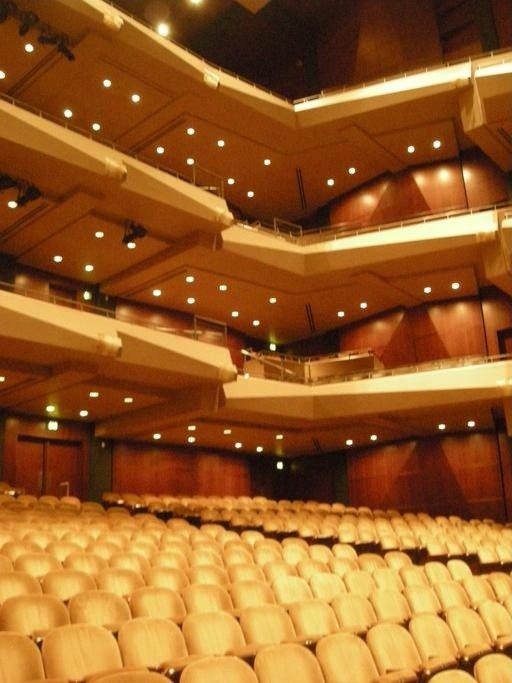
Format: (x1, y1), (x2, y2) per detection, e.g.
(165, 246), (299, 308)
(16, 187), (40, 207)
(20, 14), (37, 36)
(37, 34), (57, 49)
(58, 41), (75, 62)
(122, 227), (147, 244)
